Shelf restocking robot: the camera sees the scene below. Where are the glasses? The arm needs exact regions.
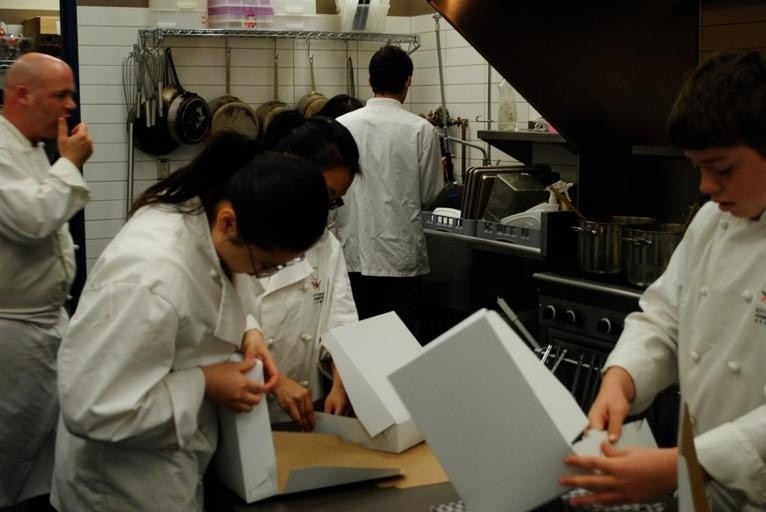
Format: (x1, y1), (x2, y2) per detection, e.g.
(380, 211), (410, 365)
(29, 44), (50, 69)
(247, 241), (306, 280)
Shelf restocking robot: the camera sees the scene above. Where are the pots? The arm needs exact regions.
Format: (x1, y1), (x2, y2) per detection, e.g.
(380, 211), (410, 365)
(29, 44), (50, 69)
(569, 210), (690, 288)
(126, 47), (367, 156)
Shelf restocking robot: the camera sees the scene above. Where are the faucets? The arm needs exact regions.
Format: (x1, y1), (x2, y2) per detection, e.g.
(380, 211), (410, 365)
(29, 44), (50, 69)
(436, 133), (487, 161)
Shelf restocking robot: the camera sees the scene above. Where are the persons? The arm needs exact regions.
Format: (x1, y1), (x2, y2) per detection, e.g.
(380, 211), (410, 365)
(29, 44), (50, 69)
(49, 130), (329, 512)
(313, 95), (364, 120)
(1, 51), (92, 512)
(247, 108), (364, 433)
(326, 45), (445, 334)
(559, 49), (766, 512)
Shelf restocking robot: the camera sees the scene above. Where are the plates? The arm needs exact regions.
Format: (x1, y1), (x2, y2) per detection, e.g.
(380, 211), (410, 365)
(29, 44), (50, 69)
(430, 206), (463, 229)
(498, 200), (553, 230)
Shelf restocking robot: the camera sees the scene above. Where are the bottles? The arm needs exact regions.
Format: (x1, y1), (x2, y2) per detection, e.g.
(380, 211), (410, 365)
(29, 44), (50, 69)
(351, 0), (371, 30)
